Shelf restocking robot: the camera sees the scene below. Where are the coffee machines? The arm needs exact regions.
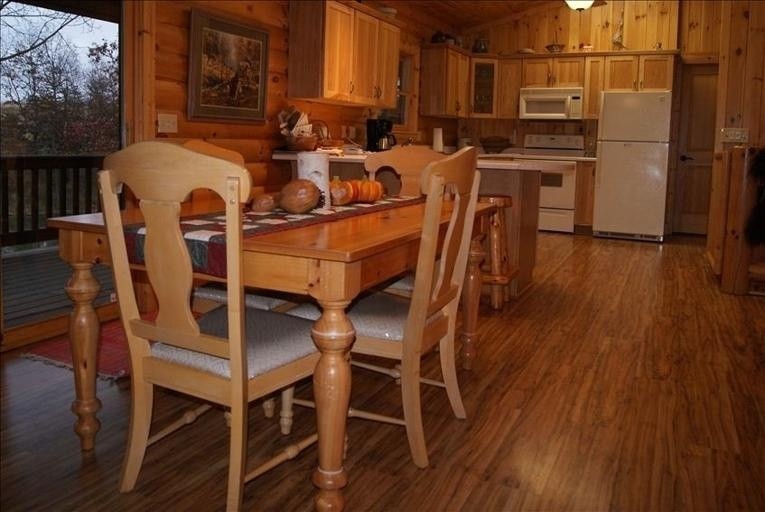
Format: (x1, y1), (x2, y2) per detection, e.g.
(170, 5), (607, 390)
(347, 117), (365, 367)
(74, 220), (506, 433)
(366, 119), (392, 152)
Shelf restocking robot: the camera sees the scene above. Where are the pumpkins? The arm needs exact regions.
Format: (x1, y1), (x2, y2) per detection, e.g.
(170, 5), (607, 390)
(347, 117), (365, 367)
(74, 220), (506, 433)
(251, 175), (384, 215)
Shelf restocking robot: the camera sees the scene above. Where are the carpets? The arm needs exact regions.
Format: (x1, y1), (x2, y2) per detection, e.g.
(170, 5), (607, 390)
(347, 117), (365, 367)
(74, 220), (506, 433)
(23, 311), (206, 381)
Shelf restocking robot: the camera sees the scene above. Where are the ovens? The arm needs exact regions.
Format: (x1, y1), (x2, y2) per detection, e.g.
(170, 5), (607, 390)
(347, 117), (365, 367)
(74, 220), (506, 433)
(511, 158), (588, 234)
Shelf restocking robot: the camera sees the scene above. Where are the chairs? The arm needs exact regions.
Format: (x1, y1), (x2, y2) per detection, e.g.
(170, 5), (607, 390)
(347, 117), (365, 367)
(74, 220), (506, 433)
(98, 143), (349, 512)
(169, 139), (293, 315)
(279, 147), (478, 469)
(363, 146), (458, 301)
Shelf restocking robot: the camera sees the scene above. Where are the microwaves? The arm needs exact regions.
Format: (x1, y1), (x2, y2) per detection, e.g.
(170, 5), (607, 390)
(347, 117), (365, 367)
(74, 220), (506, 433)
(518, 88), (584, 118)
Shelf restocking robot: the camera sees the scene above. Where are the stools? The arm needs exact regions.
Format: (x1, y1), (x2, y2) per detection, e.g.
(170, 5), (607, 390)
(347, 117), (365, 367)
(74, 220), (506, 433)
(478, 194), (520, 310)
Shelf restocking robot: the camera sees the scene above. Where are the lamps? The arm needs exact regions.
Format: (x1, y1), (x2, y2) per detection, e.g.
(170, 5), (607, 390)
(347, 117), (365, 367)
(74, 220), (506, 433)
(566, 0), (595, 12)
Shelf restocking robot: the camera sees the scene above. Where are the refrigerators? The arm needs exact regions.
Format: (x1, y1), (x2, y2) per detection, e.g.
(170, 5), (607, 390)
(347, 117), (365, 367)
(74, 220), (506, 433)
(591, 89), (681, 243)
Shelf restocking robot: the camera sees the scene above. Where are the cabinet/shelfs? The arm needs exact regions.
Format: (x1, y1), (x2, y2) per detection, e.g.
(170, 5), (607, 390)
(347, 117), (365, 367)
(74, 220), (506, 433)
(420, 42), (681, 118)
(286, 3), (353, 103)
(355, 6), (400, 110)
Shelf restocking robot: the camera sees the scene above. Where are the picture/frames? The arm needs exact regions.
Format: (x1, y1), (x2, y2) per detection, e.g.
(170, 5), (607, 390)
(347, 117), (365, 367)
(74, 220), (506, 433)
(187, 10), (270, 124)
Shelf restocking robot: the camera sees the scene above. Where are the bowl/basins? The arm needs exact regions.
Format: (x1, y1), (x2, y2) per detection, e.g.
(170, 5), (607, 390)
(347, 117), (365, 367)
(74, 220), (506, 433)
(286, 135), (316, 152)
(545, 44), (566, 52)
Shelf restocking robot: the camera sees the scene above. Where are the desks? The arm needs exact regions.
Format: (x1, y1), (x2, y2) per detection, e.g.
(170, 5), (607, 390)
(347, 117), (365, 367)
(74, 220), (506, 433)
(45, 197), (498, 511)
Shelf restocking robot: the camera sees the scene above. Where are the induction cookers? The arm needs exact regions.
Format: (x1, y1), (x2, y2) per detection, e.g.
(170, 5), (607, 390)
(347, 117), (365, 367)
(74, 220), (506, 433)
(517, 148), (596, 158)
